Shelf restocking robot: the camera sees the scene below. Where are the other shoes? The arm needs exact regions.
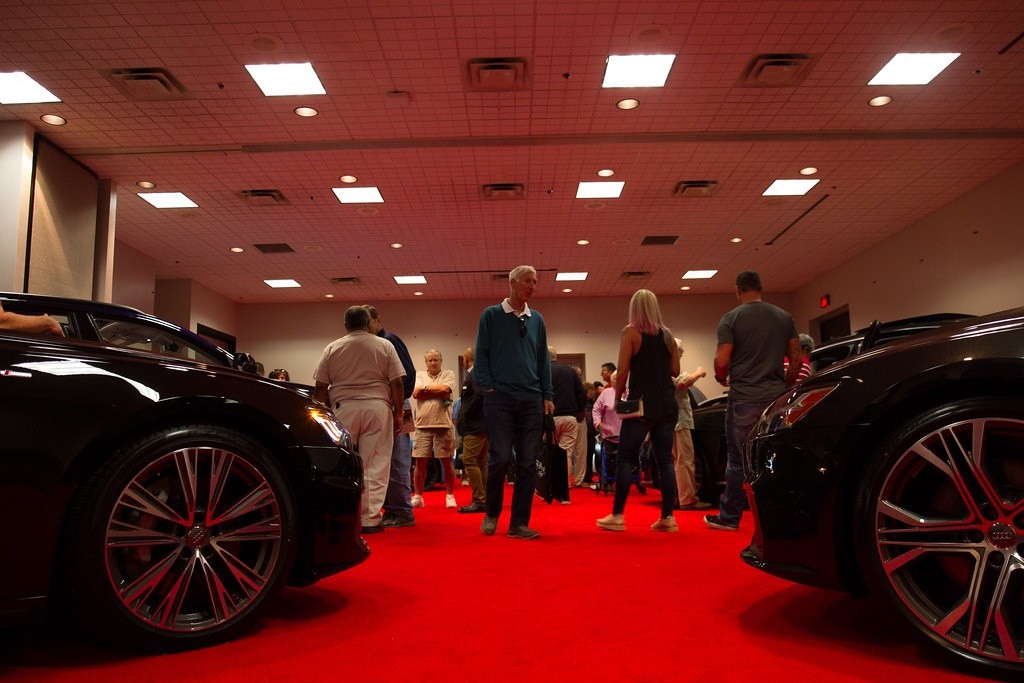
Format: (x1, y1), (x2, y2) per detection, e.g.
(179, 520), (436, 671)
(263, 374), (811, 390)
(679, 497), (711, 510)
(361, 522), (383, 533)
(576, 481), (595, 488)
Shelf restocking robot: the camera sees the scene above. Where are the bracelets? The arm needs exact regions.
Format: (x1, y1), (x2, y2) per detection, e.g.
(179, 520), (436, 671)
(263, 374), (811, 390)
(715, 374), (728, 383)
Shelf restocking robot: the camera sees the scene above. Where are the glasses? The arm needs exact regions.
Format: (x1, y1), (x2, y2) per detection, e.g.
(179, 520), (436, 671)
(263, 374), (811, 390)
(518, 315), (527, 338)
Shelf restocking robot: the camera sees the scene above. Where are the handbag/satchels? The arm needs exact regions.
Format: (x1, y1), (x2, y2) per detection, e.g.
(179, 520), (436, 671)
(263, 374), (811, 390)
(616, 398), (644, 418)
(534, 411), (571, 504)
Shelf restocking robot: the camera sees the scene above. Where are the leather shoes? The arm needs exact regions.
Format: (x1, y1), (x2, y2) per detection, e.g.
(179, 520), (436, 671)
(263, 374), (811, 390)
(457, 499), (486, 513)
(507, 523), (539, 539)
(596, 514), (626, 532)
(480, 514), (498, 534)
(651, 516), (678, 532)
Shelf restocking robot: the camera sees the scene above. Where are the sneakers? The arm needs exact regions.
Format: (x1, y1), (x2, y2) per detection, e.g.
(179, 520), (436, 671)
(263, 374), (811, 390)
(381, 509), (416, 527)
(446, 494), (457, 508)
(410, 495), (425, 510)
(704, 514), (739, 530)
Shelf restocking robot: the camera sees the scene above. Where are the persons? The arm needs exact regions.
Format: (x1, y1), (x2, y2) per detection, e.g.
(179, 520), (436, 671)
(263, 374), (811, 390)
(0, 299), (66, 337)
(784, 334), (816, 386)
(269, 369), (290, 382)
(547, 345), (586, 488)
(362, 305), (417, 528)
(596, 289), (684, 532)
(571, 363), (629, 491)
(256, 362), (264, 377)
(474, 265), (555, 538)
(312, 305), (407, 535)
(452, 346), (488, 513)
(671, 338), (706, 509)
(505, 444), (516, 484)
(704, 272), (803, 530)
(411, 349), (457, 508)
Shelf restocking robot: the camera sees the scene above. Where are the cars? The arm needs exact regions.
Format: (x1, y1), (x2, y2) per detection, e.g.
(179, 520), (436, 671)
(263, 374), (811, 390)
(0, 292), (371, 649)
(652, 314), (977, 507)
(741, 302), (1024, 683)
